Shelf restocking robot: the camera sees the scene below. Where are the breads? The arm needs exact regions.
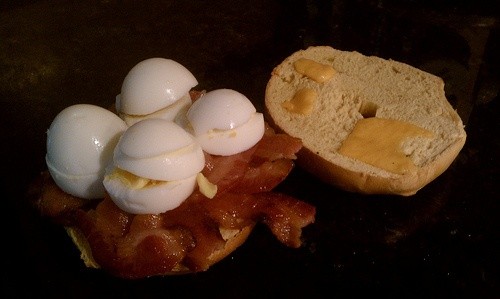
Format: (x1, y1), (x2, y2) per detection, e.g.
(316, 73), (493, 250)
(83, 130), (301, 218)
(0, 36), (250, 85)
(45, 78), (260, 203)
(64, 219), (256, 276)
(264, 46), (467, 194)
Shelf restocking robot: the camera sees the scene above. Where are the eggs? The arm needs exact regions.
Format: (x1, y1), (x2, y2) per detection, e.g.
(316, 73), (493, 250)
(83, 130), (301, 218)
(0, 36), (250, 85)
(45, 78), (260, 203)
(45, 58), (265, 213)
